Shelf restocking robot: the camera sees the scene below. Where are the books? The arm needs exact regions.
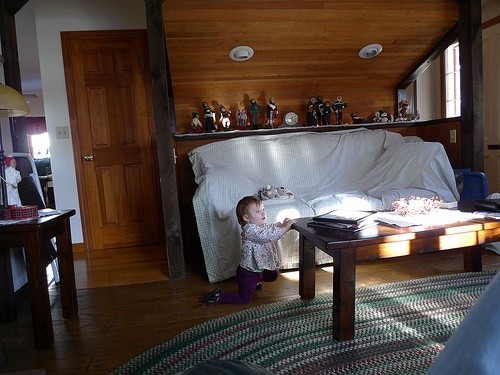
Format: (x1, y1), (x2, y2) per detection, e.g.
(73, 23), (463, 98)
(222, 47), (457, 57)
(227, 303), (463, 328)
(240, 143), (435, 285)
(306, 208), (380, 232)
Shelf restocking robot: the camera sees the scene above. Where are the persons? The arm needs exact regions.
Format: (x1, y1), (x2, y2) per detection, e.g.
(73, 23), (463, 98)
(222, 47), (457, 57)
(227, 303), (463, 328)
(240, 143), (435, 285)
(332, 95), (347, 125)
(263, 96), (278, 130)
(249, 98), (261, 130)
(219, 106), (232, 132)
(191, 112), (203, 133)
(4, 156), (21, 204)
(201, 101), (218, 133)
(235, 103), (248, 130)
(200, 195), (297, 305)
(306, 95), (333, 127)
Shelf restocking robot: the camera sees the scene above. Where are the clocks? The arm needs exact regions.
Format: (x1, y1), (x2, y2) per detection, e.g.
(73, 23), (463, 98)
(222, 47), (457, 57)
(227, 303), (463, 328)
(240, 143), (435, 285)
(282, 110), (300, 126)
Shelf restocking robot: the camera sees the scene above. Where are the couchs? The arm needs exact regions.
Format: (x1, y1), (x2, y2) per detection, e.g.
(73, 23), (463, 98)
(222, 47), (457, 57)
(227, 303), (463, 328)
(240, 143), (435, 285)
(186, 127), (489, 283)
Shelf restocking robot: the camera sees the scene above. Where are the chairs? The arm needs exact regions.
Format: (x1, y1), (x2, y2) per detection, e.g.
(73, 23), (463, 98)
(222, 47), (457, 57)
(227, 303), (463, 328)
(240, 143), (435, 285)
(457, 169), (488, 201)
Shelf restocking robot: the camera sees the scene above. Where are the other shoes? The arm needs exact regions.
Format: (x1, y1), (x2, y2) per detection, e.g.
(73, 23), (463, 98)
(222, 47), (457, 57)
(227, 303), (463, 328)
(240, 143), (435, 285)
(200, 287), (220, 302)
(256, 283), (262, 290)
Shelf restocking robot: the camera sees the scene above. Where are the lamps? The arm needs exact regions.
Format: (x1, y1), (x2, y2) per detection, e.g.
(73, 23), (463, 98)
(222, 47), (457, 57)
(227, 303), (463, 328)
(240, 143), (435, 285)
(359, 44), (382, 59)
(230, 45), (254, 63)
(0, 83), (38, 220)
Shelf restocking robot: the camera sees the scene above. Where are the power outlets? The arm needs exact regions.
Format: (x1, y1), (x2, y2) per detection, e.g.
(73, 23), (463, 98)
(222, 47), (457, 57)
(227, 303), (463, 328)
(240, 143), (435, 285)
(449, 129), (457, 143)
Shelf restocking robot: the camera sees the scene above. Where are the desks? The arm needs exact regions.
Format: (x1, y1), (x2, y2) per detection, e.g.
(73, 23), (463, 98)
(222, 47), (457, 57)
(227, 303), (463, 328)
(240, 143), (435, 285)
(0, 208), (79, 352)
(286, 207), (500, 337)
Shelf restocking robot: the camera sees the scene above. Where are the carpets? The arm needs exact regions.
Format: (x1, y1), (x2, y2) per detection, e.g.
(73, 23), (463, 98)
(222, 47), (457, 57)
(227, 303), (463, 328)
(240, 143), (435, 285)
(106, 270), (500, 375)
